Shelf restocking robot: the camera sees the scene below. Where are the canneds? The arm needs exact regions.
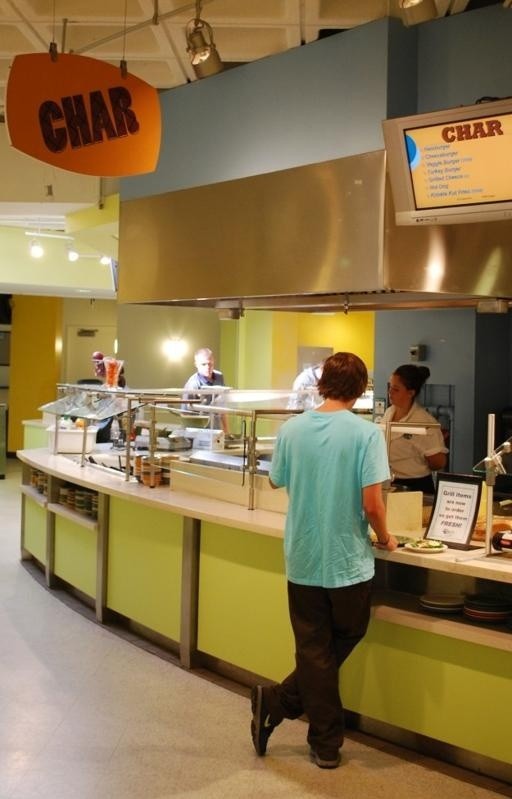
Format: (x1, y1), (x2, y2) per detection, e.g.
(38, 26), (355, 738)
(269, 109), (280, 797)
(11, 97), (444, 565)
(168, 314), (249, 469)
(29, 468), (98, 520)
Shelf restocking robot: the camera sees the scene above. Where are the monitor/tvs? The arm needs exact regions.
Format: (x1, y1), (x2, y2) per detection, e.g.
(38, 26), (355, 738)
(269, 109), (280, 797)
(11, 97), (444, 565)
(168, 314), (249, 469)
(380, 97), (512, 227)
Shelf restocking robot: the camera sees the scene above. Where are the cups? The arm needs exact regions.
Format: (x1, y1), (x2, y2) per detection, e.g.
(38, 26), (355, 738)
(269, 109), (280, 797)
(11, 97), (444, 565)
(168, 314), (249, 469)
(102, 359), (124, 390)
(134, 455), (179, 487)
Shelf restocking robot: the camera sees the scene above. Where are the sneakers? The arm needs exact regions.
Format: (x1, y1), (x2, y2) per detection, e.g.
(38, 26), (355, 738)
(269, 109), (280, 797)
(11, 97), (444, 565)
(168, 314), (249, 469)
(309, 746), (341, 768)
(250, 684), (283, 757)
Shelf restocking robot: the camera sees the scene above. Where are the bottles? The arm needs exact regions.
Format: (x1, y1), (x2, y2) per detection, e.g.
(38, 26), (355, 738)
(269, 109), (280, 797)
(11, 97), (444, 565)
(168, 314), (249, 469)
(58, 415), (85, 430)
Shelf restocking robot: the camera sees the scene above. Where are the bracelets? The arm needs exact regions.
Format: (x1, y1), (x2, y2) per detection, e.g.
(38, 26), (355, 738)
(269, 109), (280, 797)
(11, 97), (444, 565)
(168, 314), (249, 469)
(377, 533), (390, 545)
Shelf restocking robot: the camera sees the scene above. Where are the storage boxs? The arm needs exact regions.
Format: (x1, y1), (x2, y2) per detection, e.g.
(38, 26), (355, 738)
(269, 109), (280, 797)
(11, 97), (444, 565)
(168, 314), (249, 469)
(47, 423), (99, 454)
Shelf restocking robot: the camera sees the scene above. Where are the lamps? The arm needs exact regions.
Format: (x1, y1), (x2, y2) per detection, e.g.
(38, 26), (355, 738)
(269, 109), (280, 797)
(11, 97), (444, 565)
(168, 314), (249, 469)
(183, 1), (223, 78)
(398, 0), (439, 27)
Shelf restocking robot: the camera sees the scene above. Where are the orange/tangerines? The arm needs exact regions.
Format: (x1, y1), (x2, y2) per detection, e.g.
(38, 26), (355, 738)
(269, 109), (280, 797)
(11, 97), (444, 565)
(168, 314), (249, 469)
(76, 418), (87, 427)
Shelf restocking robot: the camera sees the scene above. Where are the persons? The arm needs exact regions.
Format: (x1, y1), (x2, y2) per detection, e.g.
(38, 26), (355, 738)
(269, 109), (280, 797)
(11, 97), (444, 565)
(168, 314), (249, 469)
(181, 348), (235, 440)
(251, 352), (398, 769)
(379, 364), (449, 494)
(287, 355), (329, 411)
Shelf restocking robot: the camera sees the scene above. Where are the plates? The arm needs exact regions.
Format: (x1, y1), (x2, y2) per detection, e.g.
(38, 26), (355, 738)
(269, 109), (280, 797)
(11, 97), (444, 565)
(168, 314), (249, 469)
(30, 470), (98, 519)
(418, 594), (511, 624)
(368, 532), (449, 553)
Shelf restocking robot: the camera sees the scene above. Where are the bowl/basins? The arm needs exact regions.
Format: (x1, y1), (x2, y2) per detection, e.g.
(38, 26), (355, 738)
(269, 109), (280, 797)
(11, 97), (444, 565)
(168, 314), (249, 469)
(181, 416), (210, 428)
